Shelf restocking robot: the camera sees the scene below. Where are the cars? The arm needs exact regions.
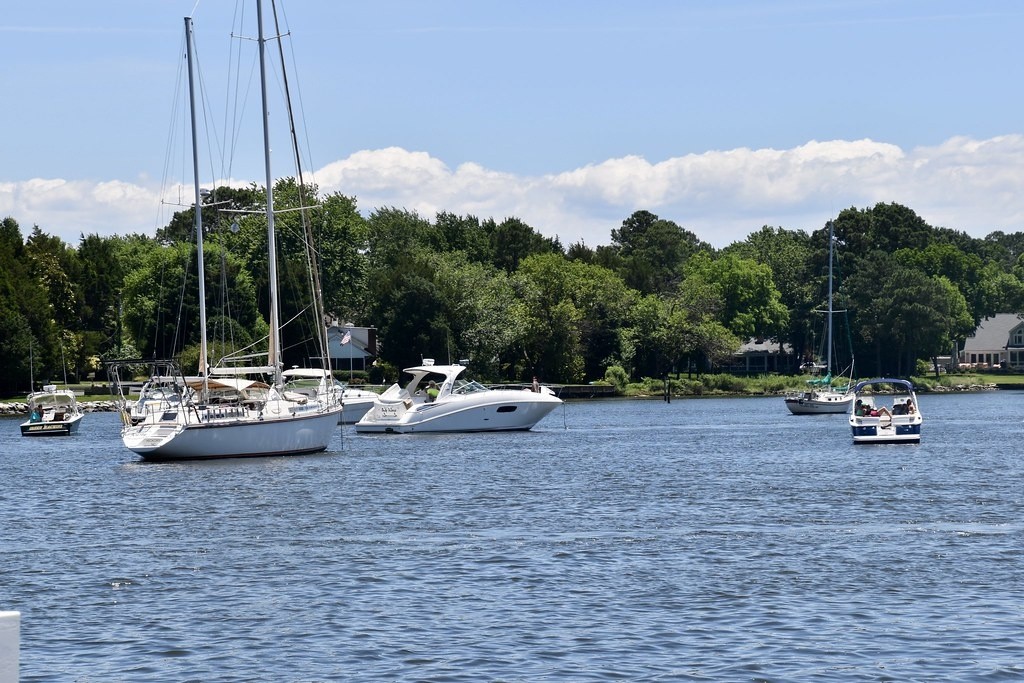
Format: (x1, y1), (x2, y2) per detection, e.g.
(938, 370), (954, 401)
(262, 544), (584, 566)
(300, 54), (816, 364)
(799, 361), (816, 373)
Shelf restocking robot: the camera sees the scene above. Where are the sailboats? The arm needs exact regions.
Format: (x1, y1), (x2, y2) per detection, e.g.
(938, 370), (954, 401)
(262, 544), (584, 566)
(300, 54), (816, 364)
(784, 217), (860, 413)
(100, 0), (380, 465)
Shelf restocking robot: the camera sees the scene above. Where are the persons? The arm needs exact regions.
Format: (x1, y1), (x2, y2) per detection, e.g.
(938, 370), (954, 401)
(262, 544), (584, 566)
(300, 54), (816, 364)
(427, 380), (439, 397)
(855, 399), (891, 417)
(899, 399), (914, 414)
(33, 405), (44, 418)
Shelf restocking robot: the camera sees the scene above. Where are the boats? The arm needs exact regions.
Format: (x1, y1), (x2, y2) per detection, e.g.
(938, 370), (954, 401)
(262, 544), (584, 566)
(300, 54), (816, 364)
(352, 357), (565, 435)
(19, 340), (86, 437)
(847, 378), (923, 444)
